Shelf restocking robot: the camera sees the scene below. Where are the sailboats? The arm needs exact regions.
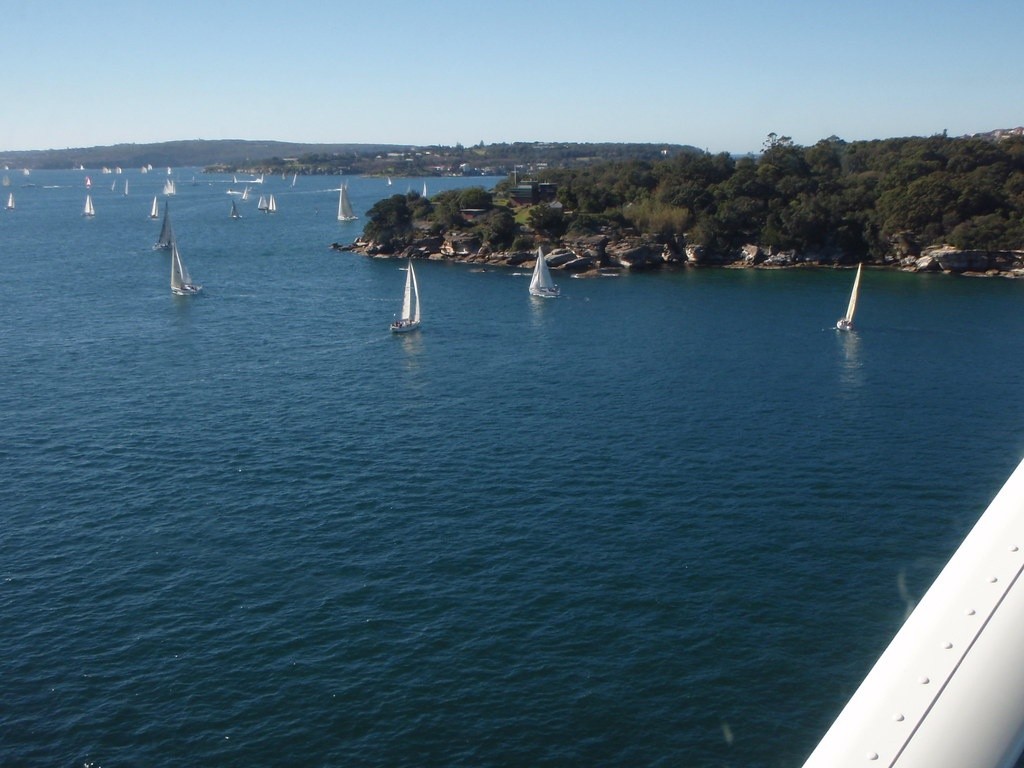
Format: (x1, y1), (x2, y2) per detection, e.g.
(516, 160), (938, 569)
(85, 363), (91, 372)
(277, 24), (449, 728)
(152, 199), (176, 251)
(1, 162), (172, 196)
(389, 257), (421, 334)
(170, 239), (203, 296)
(4, 191), (15, 211)
(224, 172), (277, 220)
(80, 191), (96, 217)
(147, 195), (160, 220)
(337, 182), (359, 222)
(531, 247), (562, 299)
(836, 262), (862, 332)
(162, 178), (178, 198)
